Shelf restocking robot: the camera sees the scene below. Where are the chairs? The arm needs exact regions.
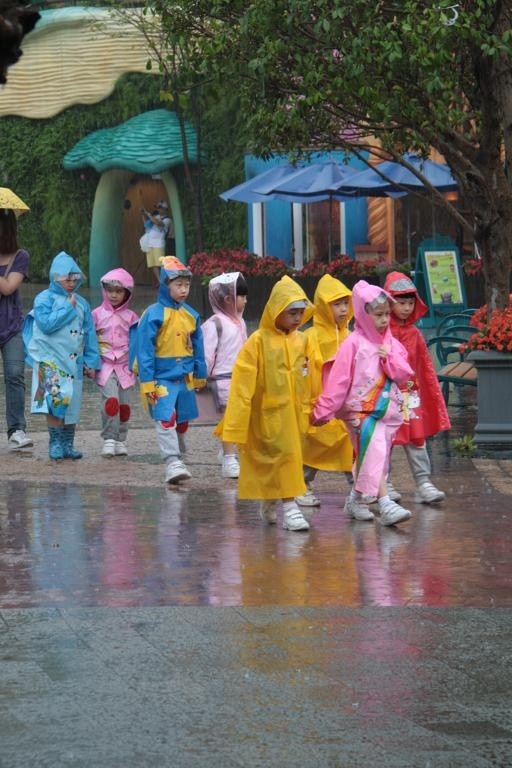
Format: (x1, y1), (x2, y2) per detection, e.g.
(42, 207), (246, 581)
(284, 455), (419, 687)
(427, 325), (478, 409)
(435, 308), (479, 395)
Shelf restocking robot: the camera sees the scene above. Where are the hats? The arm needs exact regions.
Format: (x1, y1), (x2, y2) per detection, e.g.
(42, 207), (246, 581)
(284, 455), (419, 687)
(154, 200), (170, 211)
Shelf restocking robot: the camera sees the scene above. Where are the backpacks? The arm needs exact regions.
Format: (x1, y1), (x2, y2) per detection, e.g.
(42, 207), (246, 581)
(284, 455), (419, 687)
(22, 310), (37, 368)
(129, 319), (142, 375)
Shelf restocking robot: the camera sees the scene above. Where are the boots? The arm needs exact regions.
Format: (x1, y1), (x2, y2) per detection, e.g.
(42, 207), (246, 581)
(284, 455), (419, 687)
(47, 427), (65, 460)
(62, 430), (84, 460)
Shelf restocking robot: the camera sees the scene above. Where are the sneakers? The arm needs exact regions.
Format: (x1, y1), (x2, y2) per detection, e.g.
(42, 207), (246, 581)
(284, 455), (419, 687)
(386, 483), (403, 504)
(9, 430), (34, 452)
(414, 482), (445, 504)
(114, 440), (127, 456)
(222, 458), (241, 478)
(217, 448), (239, 464)
(294, 486), (321, 508)
(343, 496), (375, 522)
(101, 439), (116, 458)
(282, 507), (312, 532)
(258, 498), (279, 525)
(349, 486), (379, 503)
(380, 506), (412, 527)
(352, 489), (378, 505)
(164, 462), (192, 482)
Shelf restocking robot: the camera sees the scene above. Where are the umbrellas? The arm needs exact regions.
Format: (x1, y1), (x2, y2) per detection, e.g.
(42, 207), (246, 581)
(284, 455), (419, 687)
(251, 155), (409, 263)
(1, 187), (31, 218)
(218, 156), (359, 262)
(331, 150), (463, 271)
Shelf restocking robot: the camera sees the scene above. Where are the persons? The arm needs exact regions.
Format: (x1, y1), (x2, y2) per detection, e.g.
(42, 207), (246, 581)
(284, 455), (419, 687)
(83, 267), (141, 459)
(128, 255), (208, 484)
(138, 201), (170, 284)
(212, 275), (325, 531)
(201, 271), (249, 479)
(0, 208), (34, 450)
(384, 271), (451, 504)
(294, 273), (378, 507)
(309, 280), (416, 526)
(22, 252), (102, 462)
